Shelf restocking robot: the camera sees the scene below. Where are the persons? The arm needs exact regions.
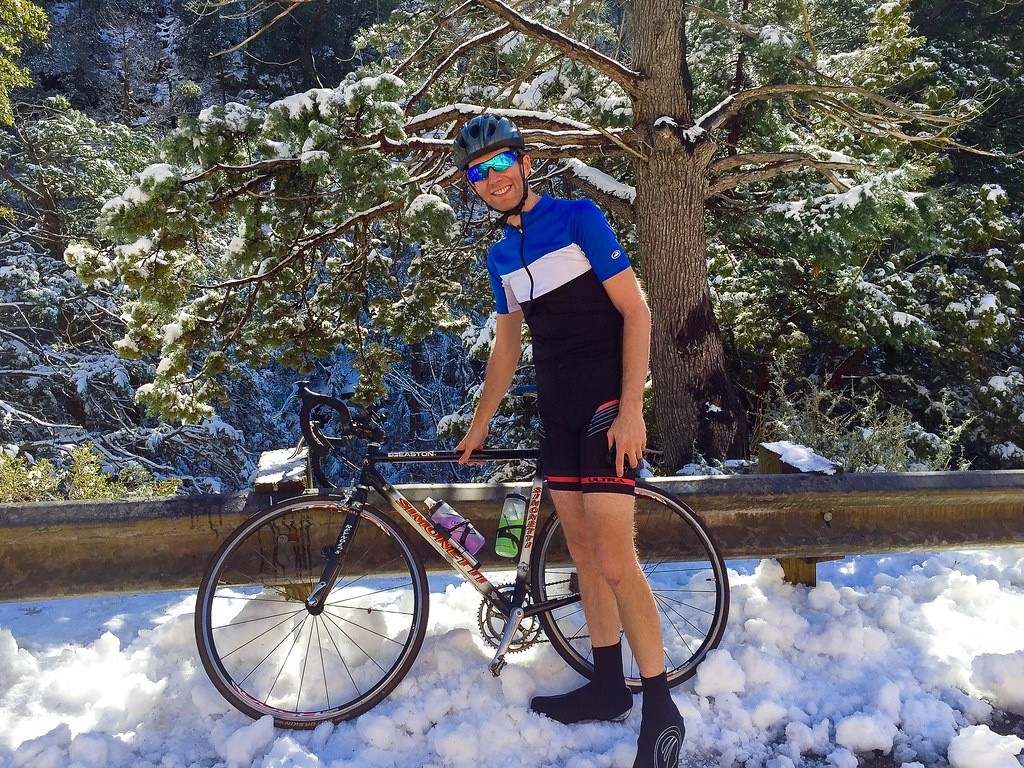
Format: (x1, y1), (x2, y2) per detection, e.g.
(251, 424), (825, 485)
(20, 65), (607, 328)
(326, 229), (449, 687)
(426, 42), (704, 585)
(452, 112), (687, 768)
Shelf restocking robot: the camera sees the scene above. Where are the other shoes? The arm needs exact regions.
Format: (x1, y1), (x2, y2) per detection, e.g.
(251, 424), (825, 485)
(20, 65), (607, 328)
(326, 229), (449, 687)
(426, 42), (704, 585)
(530, 680), (634, 722)
(632, 712), (685, 768)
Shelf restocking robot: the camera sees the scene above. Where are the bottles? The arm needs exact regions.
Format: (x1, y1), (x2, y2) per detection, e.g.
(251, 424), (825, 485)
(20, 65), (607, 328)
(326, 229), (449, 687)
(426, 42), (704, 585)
(495, 485), (528, 557)
(424, 496), (485, 556)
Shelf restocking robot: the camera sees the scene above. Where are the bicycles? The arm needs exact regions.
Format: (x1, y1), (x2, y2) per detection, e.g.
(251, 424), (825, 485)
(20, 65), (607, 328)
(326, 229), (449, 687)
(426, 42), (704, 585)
(194, 377), (732, 730)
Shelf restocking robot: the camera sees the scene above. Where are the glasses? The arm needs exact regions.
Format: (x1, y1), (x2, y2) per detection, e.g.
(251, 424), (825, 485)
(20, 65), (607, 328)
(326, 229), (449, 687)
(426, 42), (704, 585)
(466, 150), (518, 182)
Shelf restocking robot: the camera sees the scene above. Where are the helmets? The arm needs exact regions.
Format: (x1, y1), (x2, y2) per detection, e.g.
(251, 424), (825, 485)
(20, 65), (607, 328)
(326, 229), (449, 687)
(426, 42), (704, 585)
(452, 112), (524, 173)
